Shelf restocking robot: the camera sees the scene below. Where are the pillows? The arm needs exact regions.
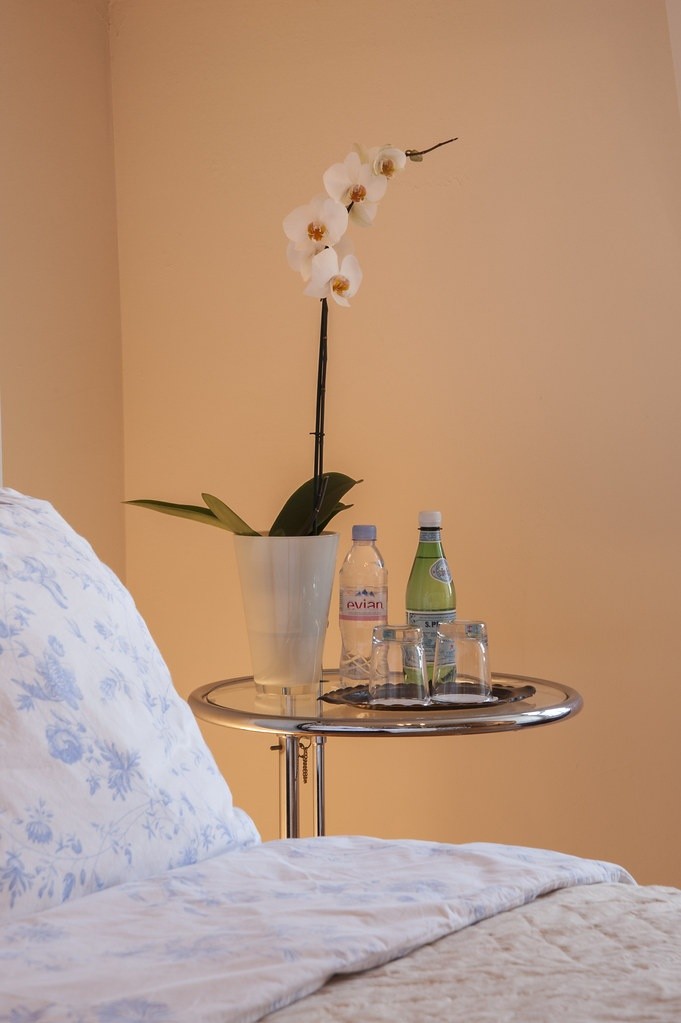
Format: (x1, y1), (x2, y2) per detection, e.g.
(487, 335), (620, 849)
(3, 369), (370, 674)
(0, 485), (261, 930)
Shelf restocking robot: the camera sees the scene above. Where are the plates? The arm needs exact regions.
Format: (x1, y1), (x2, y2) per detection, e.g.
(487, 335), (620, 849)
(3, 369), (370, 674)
(316, 682), (536, 711)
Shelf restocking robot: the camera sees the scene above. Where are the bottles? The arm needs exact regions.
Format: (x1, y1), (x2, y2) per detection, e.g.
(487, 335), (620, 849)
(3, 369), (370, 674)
(339, 525), (389, 688)
(402, 511), (457, 685)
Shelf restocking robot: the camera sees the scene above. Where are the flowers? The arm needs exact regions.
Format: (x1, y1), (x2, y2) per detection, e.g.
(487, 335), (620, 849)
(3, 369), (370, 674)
(116, 138), (462, 535)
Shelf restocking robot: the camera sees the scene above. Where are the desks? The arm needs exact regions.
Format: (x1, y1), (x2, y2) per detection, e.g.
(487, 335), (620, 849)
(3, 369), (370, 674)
(189, 668), (582, 840)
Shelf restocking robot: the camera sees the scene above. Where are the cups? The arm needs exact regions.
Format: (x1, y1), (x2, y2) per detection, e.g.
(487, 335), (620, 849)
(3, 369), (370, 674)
(369, 625), (430, 704)
(432, 620), (495, 703)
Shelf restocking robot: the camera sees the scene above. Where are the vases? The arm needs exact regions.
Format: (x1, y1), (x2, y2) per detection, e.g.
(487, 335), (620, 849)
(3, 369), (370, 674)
(232, 531), (341, 686)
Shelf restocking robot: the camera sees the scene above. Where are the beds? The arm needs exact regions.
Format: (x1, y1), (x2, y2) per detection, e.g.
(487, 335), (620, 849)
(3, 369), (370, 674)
(0, 837), (680, 1022)
